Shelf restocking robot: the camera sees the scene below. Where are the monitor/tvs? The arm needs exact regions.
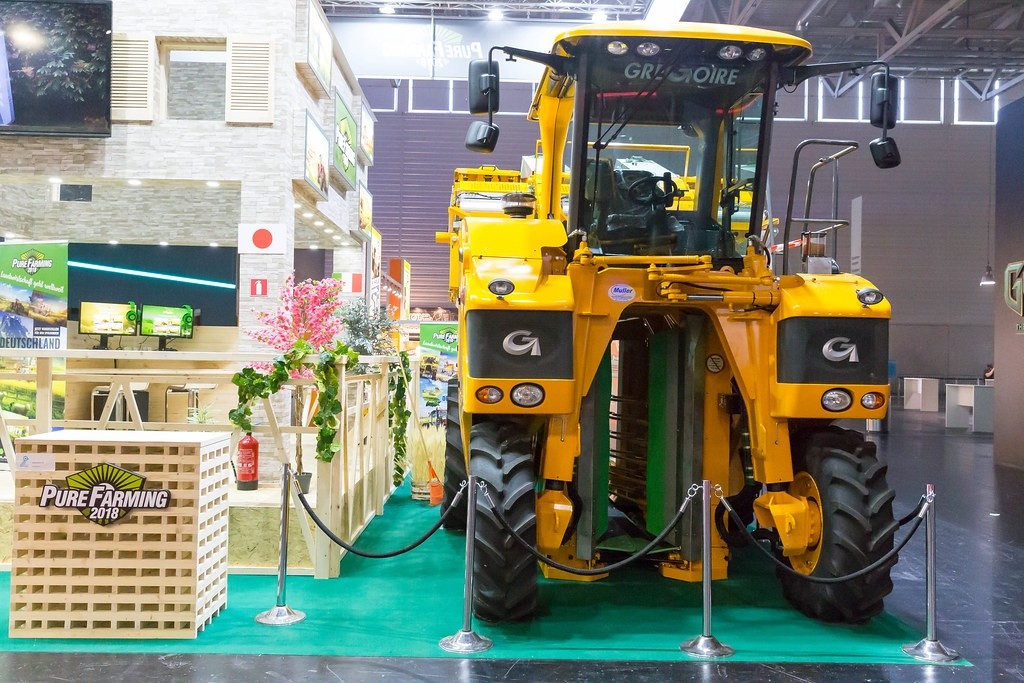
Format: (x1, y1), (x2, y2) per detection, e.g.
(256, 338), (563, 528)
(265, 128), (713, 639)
(78, 301), (138, 350)
(140, 304), (194, 351)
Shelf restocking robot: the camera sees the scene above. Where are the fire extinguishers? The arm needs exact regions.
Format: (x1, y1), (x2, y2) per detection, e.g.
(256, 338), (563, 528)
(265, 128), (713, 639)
(230, 432), (260, 490)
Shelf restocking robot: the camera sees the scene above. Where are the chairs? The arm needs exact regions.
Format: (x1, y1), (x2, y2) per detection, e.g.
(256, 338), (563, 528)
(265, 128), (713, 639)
(606, 170), (667, 236)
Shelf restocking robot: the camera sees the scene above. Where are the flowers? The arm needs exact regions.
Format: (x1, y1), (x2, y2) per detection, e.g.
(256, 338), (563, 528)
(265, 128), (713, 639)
(339, 295), (400, 379)
(242, 271), (345, 473)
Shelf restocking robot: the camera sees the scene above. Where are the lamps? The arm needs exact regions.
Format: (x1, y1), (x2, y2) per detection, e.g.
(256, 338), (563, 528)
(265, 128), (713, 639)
(980, 98), (996, 286)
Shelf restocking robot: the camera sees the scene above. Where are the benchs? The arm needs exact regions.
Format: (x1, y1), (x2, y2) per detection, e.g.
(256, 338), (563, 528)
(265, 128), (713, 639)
(897, 374), (983, 400)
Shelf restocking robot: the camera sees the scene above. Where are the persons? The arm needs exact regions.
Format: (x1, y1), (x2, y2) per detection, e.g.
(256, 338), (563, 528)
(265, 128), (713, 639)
(984, 363), (994, 384)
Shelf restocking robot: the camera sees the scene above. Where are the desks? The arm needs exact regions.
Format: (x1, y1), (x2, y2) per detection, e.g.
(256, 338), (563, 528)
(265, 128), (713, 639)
(945, 383), (993, 432)
(984, 379), (995, 386)
(904, 378), (939, 411)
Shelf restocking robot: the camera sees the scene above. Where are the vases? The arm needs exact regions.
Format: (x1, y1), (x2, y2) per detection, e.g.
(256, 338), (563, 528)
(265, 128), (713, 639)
(294, 472), (312, 493)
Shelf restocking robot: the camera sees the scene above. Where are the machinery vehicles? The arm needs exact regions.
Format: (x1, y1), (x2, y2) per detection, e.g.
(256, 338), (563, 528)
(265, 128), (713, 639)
(433, 18), (903, 624)
